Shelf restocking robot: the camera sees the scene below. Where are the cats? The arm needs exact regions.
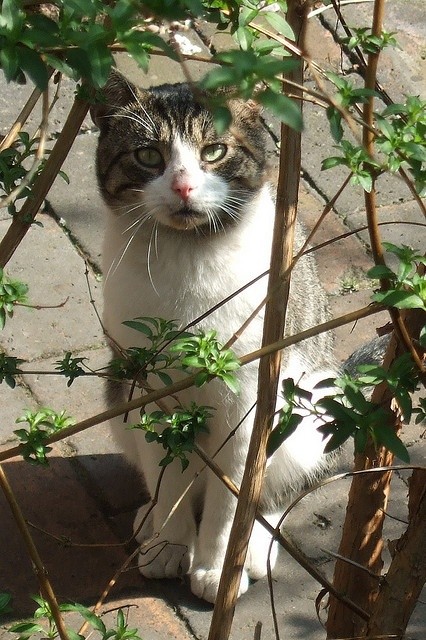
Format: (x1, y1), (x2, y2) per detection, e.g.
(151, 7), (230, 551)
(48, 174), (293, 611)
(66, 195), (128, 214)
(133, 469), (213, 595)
(84, 66), (391, 607)
